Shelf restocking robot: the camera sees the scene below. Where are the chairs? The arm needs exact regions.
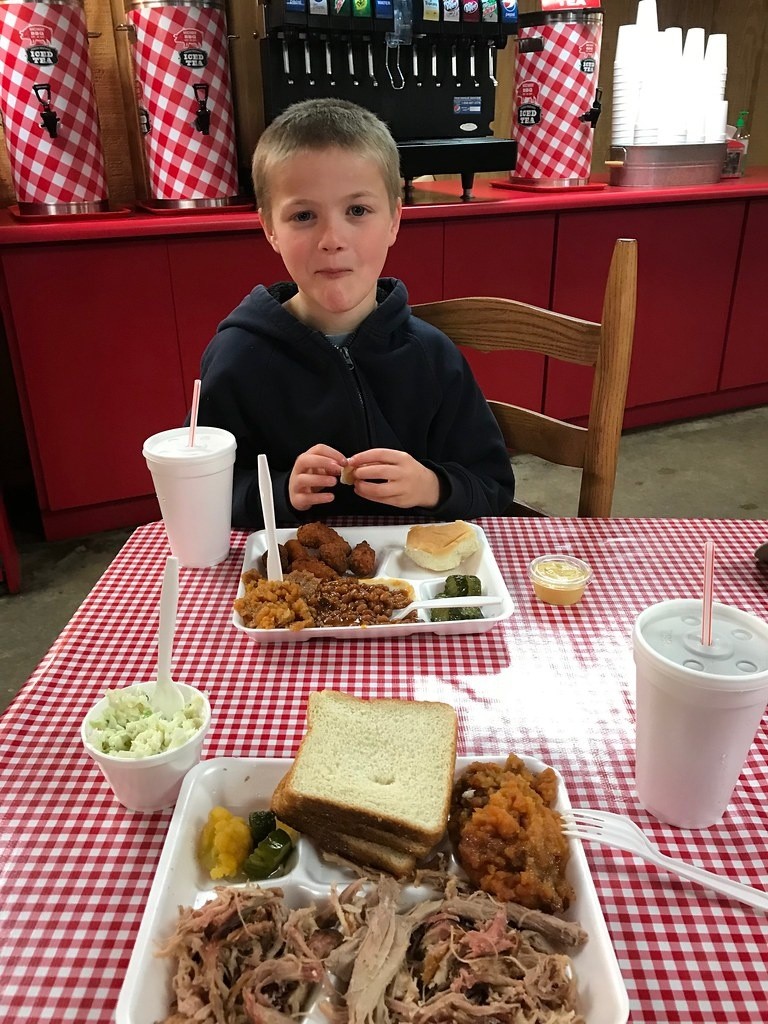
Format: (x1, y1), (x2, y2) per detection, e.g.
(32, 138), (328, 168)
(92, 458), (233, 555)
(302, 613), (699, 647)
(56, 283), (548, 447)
(407, 238), (639, 517)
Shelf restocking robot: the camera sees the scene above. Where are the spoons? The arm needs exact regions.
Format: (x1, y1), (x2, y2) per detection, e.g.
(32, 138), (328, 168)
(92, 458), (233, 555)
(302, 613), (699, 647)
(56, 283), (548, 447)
(559, 810), (768, 920)
(150, 556), (184, 721)
(388, 595), (503, 621)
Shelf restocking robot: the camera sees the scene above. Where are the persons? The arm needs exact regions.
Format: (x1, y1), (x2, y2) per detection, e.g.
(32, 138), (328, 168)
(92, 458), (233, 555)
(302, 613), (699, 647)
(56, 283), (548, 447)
(181, 98), (516, 527)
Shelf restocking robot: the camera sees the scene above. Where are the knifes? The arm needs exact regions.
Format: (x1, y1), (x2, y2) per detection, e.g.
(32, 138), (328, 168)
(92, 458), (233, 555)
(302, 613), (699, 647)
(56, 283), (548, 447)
(258, 454), (282, 581)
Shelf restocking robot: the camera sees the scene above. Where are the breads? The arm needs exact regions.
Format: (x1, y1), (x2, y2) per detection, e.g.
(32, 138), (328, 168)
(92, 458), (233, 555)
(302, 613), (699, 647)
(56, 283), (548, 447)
(272, 688), (459, 877)
(405, 520), (480, 571)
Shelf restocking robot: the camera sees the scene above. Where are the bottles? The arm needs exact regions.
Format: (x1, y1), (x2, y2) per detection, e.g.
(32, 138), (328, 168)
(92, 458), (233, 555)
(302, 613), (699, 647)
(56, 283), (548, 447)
(732, 111), (751, 176)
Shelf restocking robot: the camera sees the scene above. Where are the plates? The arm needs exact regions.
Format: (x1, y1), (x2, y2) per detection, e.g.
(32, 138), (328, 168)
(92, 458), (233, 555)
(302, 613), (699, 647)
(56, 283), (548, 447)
(230, 522), (516, 640)
(111, 755), (628, 1024)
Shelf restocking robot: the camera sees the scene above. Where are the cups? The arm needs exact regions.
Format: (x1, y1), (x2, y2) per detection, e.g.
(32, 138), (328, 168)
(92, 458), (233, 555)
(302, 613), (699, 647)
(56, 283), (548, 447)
(141, 426), (237, 568)
(611, 0), (729, 145)
(629, 596), (767, 830)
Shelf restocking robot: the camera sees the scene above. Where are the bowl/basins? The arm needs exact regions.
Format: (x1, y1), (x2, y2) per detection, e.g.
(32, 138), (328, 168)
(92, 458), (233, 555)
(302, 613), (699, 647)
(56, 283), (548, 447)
(80, 680), (211, 811)
(528, 555), (594, 605)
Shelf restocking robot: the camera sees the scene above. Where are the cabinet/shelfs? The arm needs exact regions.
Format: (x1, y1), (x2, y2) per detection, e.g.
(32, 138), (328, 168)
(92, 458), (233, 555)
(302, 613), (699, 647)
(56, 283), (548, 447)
(0, 165), (768, 543)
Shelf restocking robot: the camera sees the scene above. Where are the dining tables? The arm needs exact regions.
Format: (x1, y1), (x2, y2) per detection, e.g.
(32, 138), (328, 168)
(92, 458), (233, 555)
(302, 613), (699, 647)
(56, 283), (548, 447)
(0, 515), (768, 1024)
